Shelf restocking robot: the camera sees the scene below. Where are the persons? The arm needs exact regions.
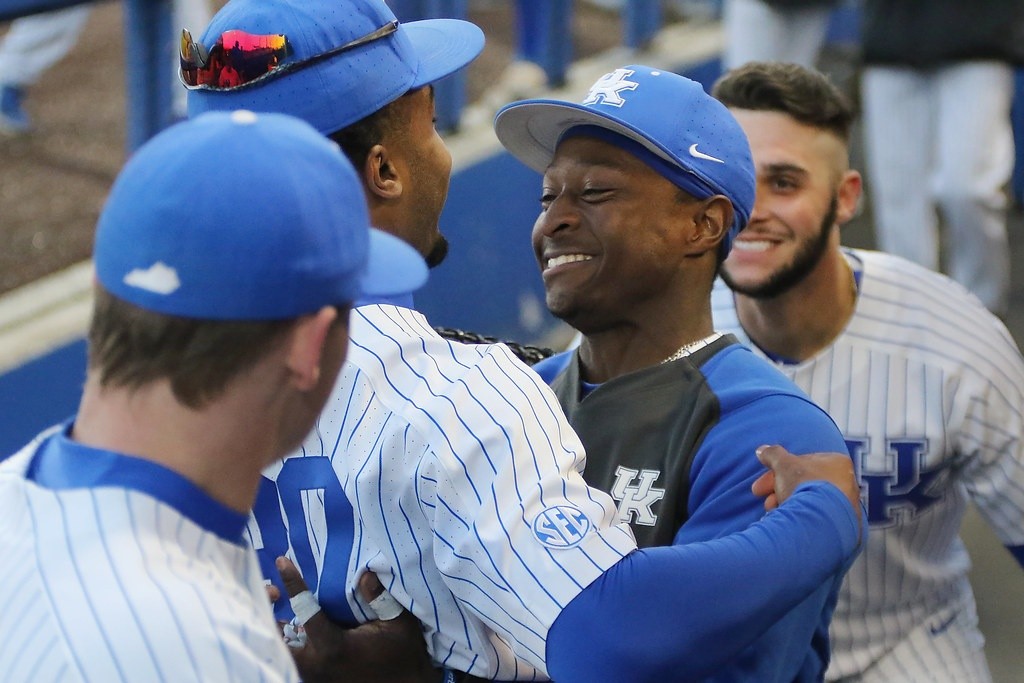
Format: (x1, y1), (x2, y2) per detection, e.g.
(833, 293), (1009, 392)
(719, 0), (1022, 324)
(264, 58), (873, 682)
(180, 0), (865, 682)
(704, 60), (1024, 683)
(1, 109), (374, 682)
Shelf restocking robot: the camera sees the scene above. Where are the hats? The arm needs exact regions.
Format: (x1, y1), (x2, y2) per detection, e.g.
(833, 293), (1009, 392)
(186, 1), (486, 139)
(494, 63), (756, 248)
(93, 111), (429, 317)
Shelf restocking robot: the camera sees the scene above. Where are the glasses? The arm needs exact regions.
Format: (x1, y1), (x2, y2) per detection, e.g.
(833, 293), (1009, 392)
(177, 21), (409, 96)
